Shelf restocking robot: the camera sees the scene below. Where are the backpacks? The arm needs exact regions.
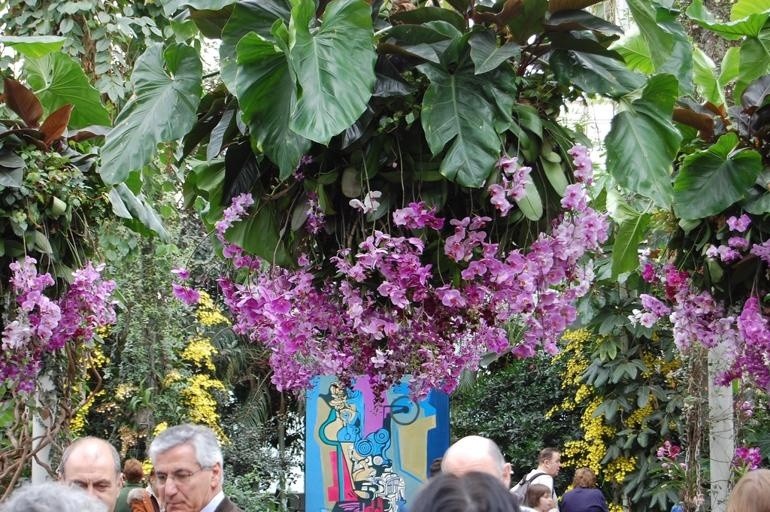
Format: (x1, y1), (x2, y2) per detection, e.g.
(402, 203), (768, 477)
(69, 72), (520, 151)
(512, 472), (554, 506)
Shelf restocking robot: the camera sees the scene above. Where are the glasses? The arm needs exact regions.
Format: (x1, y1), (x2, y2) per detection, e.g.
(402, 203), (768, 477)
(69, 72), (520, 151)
(155, 467), (204, 484)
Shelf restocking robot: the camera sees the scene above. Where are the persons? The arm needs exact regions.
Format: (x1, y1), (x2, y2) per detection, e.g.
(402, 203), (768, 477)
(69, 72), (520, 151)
(672, 468), (769, 510)
(410, 435), (610, 510)
(1, 420), (242, 511)
